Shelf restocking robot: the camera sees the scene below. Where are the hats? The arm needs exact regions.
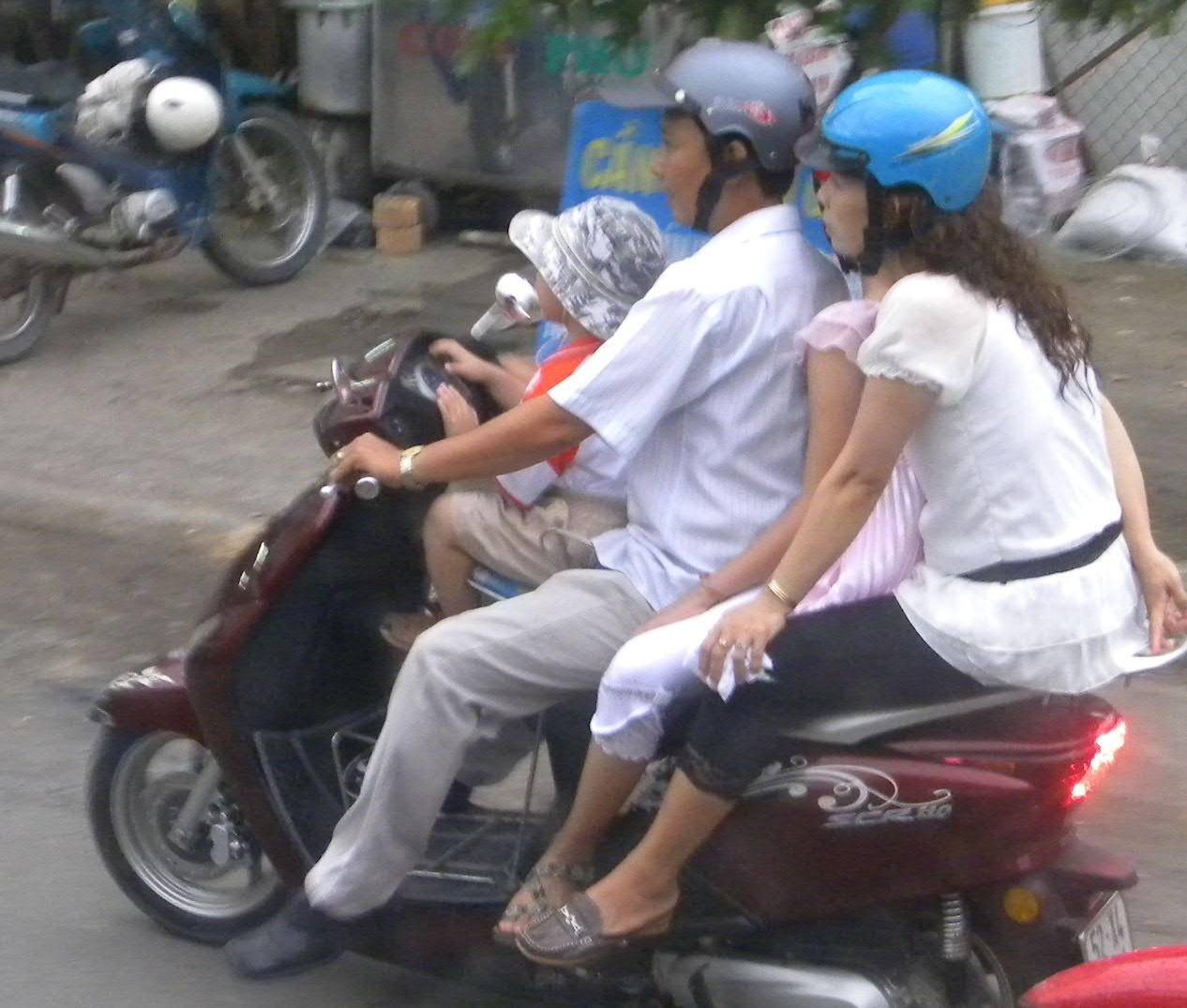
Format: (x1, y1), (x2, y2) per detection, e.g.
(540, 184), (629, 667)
(510, 194), (670, 341)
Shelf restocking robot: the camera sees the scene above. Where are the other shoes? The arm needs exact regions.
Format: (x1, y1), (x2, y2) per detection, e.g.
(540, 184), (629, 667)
(379, 610), (441, 652)
(517, 893), (673, 962)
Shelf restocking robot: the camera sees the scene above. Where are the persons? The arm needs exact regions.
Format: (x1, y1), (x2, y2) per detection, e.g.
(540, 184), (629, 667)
(513, 69), (1187, 967)
(224, 38), (862, 983)
(380, 194), (670, 653)
(495, 248), (903, 946)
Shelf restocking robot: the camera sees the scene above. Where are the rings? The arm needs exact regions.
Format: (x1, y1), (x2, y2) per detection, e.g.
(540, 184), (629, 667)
(333, 451), (345, 466)
(718, 638), (731, 648)
(735, 643), (748, 649)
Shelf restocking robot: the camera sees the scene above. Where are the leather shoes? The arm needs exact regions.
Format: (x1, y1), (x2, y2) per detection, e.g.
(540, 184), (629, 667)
(224, 885), (377, 981)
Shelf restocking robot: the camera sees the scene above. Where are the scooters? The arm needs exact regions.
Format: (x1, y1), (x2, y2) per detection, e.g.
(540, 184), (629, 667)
(85, 271), (1138, 1008)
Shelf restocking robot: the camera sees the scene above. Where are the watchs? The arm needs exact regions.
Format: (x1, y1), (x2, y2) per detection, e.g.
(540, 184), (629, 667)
(400, 446), (429, 489)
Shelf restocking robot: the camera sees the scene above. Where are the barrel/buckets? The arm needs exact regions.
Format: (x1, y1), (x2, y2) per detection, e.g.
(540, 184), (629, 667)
(955, 0), (1084, 201)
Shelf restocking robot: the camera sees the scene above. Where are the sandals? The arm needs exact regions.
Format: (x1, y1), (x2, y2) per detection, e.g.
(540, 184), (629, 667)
(493, 857), (598, 951)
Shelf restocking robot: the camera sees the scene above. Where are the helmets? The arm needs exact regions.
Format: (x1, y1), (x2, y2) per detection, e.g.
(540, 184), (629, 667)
(146, 77), (223, 150)
(795, 68), (994, 216)
(595, 38), (818, 174)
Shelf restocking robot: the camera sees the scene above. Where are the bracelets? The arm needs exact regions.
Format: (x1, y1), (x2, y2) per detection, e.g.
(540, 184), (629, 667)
(767, 577), (799, 608)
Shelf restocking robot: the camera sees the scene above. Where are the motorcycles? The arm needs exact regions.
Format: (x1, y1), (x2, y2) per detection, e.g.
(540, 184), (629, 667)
(1, 0), (330, 365)
